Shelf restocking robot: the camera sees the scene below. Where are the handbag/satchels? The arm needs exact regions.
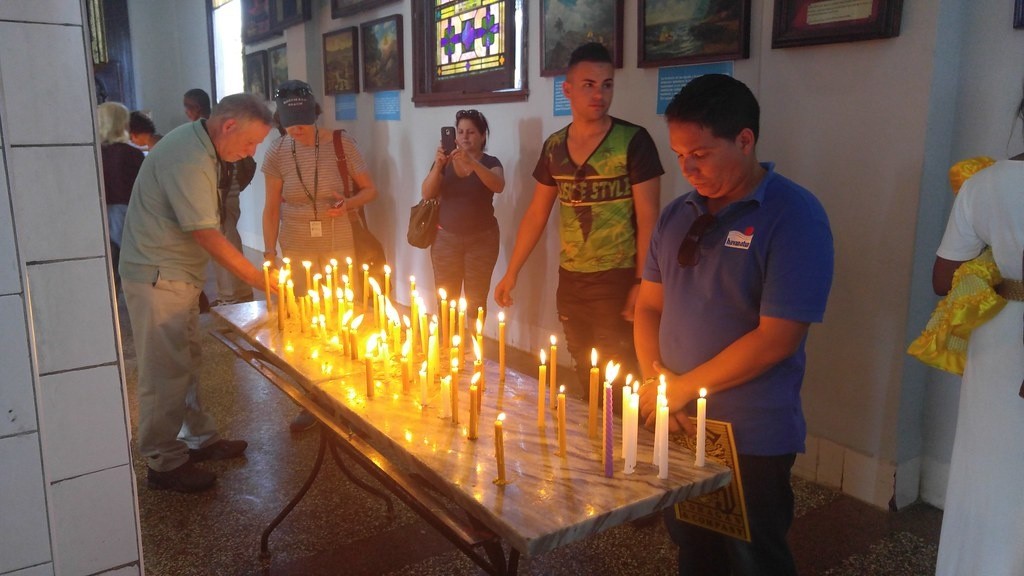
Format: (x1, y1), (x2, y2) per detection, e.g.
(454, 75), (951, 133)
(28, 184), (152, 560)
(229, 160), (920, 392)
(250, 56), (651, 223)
(407, 199), (440, 249)
(353, 226), (393, 300)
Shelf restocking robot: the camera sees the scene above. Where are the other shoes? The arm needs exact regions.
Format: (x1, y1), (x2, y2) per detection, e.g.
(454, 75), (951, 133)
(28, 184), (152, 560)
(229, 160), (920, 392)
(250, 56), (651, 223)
(209, 294), (254, 311)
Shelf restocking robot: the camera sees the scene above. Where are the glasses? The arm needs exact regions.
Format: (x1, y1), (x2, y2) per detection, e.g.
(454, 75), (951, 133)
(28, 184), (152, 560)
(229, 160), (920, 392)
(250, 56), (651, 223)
(676, 212), (717, 267)
(272, 87), (314, 102)
(456, 109), (490, 137)
(570, 166), (586, 203)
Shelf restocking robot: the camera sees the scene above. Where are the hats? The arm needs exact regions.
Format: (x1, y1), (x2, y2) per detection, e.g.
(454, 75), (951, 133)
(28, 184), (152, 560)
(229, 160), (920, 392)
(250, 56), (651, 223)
(276, 79), (317, 130)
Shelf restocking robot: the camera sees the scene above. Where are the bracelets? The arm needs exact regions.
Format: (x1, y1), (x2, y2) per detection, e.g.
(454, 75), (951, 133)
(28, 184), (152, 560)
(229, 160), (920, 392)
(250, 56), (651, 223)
(641, 376), (655, 386)
(635, 277), (643, 284)
(264, 251), (278, 259)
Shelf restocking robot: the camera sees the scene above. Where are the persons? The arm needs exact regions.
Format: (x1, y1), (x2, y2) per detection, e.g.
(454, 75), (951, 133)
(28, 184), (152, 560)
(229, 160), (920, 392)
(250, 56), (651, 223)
(261, 79), (377, 302)
(119, 92), (274, 492)
(126, 110), (210, 314)
(932, 97), (1024, 576)
(493, 42), (664, 427)
(183, 88), (254, 308)
(421, 109), (506, 362)
(96, 101), (146, 303)
(634, 73), (832, 576)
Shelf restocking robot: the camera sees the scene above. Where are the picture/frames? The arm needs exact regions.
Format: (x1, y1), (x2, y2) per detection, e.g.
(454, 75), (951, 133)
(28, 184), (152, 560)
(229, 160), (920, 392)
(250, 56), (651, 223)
(634, 0), (752, 71)
(360, 13), (405, 94)
(770, 1), (904, 49)
(320, 25), (358, 96)
(537, 0), (625, 76)
(331, 0), (401, 19)
(239, 0), (313, 105)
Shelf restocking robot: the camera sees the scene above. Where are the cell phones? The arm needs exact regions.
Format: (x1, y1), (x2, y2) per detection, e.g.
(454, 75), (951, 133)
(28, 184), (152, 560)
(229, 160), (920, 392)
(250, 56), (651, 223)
(331, 199), (344, 208)
(441, 127), (456, 155)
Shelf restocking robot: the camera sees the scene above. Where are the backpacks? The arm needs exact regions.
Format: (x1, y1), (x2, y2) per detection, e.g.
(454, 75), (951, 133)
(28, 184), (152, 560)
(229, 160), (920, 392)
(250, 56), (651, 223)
(236, 156), (256, 192)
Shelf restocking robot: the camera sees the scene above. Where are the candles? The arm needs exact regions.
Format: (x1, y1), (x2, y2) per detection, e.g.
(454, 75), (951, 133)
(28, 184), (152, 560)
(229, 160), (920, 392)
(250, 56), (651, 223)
(262, 254), (706, 486)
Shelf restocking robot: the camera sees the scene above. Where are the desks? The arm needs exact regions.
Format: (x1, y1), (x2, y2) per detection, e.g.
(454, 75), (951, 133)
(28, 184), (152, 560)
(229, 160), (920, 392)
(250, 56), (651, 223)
(206, 300), (733, 575)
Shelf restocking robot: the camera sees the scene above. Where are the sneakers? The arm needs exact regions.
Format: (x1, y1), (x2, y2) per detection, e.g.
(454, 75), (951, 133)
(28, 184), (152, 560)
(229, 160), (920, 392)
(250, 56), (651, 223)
(290, 409), (318, 432)
(148, 460), (218, 494)
(188, 438), (248, 462)
